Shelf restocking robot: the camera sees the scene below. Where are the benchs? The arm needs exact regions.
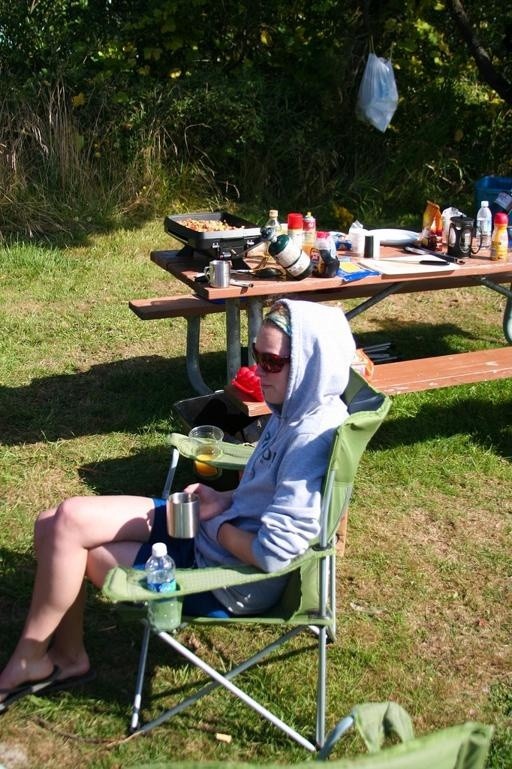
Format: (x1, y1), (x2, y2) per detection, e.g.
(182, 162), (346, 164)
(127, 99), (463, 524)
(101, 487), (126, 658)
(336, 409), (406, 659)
(128, 278), (511, 397)
(226, 346), (511, 557)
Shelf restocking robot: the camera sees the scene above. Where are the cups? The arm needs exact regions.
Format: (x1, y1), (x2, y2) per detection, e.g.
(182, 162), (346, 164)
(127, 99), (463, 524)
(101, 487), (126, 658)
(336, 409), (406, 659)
(167, 492), (199, 538)
(449, 215), (481, 255)
(204, 260), (231, 288)
(189, 423), (223, 475)
(349, 229), (366, 252)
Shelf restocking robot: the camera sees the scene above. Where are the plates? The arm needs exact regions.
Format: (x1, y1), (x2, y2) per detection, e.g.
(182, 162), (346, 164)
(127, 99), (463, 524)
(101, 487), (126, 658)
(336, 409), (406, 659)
(368, 229), (420, 247)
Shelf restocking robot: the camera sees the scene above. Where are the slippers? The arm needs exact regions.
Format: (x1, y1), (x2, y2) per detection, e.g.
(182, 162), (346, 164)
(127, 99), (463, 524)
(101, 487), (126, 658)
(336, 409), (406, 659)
(0, 662), (97, 712)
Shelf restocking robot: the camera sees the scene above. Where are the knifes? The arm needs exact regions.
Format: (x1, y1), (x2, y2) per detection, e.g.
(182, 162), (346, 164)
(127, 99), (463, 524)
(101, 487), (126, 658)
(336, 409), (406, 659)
(367, 256), (451, 265)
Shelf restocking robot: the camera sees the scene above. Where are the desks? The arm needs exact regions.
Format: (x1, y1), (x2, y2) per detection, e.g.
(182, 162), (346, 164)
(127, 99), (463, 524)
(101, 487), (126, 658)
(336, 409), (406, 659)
(150, 236), (512, 393)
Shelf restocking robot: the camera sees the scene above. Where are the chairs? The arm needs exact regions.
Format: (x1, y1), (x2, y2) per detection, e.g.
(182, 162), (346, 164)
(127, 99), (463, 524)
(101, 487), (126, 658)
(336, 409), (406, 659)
(97, 365), (392, 752)
(122, 701), (495, 769)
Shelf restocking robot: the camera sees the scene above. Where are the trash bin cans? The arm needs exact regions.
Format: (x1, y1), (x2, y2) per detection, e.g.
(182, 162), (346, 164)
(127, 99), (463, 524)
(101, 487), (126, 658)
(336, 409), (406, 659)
(473, 176), (512, 248)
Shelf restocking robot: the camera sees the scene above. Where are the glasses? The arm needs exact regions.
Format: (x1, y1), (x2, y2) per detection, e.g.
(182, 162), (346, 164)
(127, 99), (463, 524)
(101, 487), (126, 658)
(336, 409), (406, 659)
(252, 343), (290, 373)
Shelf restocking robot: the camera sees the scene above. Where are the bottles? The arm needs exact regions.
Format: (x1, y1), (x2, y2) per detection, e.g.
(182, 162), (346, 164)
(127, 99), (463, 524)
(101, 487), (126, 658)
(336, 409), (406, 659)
(262, 226), (314, 281)
(476, 202), (491, 248)
(265, 210), (282, 239)
(490, 212), (509, 261)
(146, 541), (177, 632)
(287, 211), (317, 251)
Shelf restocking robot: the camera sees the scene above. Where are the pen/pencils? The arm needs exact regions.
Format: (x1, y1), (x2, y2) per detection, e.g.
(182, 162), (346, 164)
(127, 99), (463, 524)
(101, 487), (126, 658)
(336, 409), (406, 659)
(419, 261), (450, 265)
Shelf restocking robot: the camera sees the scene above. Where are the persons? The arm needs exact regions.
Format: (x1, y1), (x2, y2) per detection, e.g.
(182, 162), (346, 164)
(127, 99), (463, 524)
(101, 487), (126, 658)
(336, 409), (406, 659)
(0, 298), (356, 713)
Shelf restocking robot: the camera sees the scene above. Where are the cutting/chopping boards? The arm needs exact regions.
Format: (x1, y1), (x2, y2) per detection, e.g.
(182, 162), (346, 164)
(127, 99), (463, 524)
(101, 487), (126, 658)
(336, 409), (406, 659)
(357, 254), (458, 275)
